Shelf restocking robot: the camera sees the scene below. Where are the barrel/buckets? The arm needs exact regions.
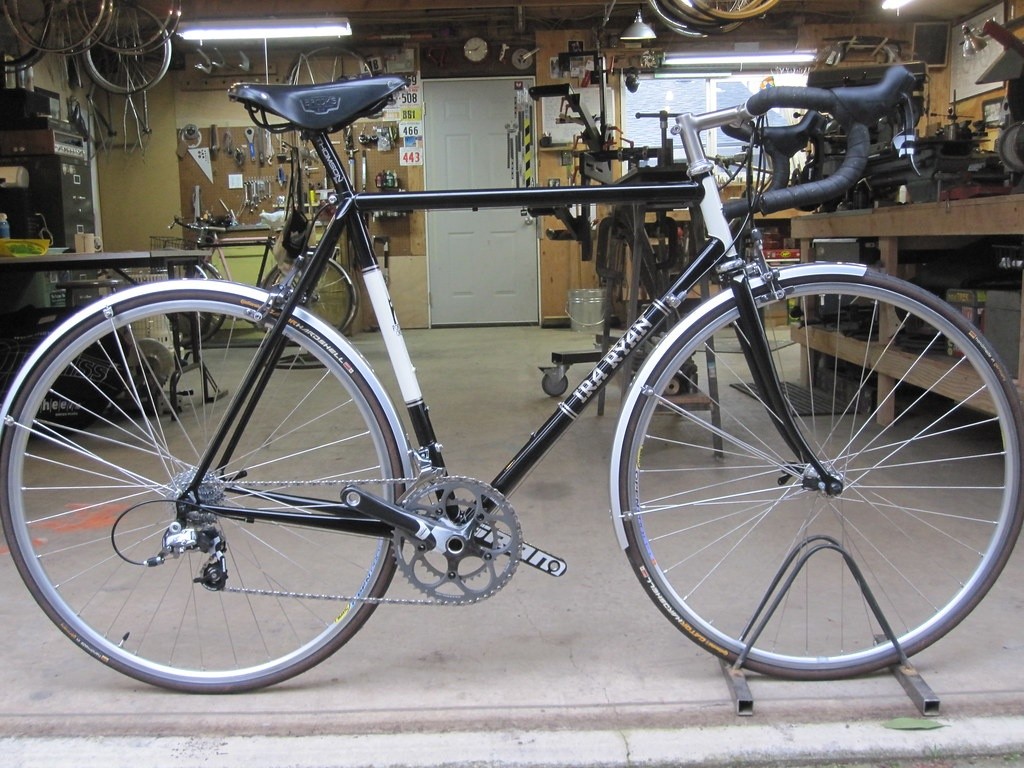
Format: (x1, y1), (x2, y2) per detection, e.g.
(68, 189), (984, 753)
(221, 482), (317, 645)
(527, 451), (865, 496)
(565, 288), (605, 335)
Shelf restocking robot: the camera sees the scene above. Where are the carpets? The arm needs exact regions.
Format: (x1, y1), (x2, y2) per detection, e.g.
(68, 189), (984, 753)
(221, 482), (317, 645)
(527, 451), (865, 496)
(731, 379), (860, 418)
(696, 337), (794, 354)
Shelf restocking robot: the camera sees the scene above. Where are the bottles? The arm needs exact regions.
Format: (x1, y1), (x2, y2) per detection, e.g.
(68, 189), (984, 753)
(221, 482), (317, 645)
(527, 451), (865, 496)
(894, 180), (911, 205)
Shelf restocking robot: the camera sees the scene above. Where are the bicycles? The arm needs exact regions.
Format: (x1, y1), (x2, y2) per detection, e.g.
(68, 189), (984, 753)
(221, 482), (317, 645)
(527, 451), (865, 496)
(150, 217), (359, 346)
(1, 62), (1024, 701)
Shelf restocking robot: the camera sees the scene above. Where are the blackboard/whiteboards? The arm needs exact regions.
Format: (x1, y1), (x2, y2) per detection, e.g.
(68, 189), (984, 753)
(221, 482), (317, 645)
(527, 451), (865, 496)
(541, 87), (612, 143)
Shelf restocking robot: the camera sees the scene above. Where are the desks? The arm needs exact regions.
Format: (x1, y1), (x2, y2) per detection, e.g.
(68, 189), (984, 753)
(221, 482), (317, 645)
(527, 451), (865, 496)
(0, 249), (229, 423)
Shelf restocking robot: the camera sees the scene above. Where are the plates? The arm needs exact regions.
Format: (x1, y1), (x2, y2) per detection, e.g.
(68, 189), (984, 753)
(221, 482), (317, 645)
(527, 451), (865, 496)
(47, 248), (69, 253)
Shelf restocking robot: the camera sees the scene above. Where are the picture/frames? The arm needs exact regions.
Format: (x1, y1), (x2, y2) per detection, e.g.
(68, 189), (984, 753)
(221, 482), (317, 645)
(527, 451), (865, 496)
(981, 96), (1005, 129)
(948, 0), (1010, 104)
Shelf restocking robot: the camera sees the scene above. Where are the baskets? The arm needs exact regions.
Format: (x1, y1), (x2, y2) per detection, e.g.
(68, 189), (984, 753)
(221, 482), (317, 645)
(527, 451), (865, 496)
(150, 234), (195, 273)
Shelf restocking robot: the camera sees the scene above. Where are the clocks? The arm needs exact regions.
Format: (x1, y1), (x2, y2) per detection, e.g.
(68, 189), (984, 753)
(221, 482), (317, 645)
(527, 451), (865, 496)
(464, 37), (487, 62)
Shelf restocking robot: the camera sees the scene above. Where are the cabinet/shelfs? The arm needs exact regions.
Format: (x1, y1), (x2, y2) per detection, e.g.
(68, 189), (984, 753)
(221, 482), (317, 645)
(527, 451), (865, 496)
(787, 190), (1024, 430)
(0, 154), (100, 307)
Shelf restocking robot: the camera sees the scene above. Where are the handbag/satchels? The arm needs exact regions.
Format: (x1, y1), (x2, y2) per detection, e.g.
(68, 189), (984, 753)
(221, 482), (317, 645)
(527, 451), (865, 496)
(273, 210), (315, 281)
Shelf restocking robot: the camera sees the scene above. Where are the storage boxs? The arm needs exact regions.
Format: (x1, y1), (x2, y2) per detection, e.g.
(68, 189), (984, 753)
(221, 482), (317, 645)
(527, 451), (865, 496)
(944, 285), (986, 366)
(74, 233), (95, 253)
(984, 291), (1021, 380)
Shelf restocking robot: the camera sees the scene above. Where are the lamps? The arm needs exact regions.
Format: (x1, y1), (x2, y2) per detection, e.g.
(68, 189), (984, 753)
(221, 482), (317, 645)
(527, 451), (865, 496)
(175, 16), (353, 42)
(959, 23), (989, 59)
(618, 9), (657, 43)
(658, 50), (820, 68)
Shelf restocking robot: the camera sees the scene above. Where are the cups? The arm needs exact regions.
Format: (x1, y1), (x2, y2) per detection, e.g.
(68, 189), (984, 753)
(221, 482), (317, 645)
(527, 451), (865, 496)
(75, 234), (103, 253)
